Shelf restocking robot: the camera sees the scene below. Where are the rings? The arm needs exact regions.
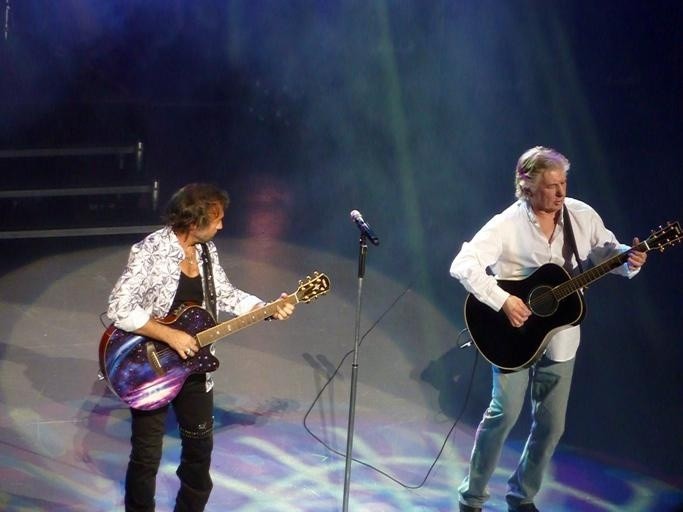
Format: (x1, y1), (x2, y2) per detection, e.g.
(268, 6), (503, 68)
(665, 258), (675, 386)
(187, 349), (191, 355)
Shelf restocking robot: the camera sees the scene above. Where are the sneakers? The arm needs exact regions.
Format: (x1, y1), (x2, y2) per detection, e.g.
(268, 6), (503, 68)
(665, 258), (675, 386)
(509, 502), (539, 512)
(459, 502), (481, 512)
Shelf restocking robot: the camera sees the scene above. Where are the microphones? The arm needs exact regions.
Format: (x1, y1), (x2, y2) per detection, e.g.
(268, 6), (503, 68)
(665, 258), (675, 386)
(350, 209), (380, 245)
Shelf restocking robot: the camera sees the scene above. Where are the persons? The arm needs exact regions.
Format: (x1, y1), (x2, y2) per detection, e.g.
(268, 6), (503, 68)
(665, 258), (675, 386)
(450, 145), (647, 512)
(106, 183), (297, 512)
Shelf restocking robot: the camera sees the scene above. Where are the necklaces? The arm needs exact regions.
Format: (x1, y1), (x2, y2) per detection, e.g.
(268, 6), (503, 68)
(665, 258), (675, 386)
(185, 247), (195, 264)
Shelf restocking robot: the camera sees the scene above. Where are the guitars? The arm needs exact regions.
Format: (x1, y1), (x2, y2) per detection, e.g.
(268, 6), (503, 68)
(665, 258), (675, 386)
(463, 217), (683, 373)
(98, 268), (331, 412)
